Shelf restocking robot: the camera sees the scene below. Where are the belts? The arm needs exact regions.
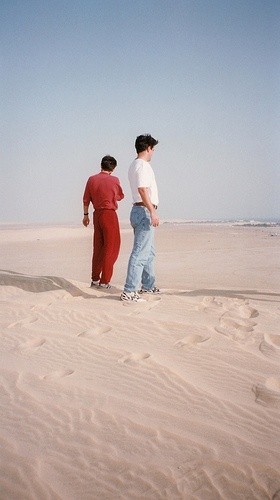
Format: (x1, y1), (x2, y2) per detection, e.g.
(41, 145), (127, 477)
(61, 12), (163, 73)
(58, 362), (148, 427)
(133, 202), (158, 210)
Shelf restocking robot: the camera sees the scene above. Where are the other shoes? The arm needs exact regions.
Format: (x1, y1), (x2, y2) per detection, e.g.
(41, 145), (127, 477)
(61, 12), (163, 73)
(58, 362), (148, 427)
(121, 291), (148, 303)
(99, 283), (116, 290)
(90, 280), (100, 288)
(139, 287), (163, 295)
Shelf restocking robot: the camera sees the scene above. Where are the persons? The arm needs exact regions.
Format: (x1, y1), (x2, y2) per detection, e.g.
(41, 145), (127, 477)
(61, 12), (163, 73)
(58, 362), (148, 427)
(82, 155), (125, 289)
(120, 134), (159, 301)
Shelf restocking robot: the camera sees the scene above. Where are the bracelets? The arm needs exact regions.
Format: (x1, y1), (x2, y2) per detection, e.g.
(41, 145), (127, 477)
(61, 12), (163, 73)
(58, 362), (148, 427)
(84, 212), (89, 215)
